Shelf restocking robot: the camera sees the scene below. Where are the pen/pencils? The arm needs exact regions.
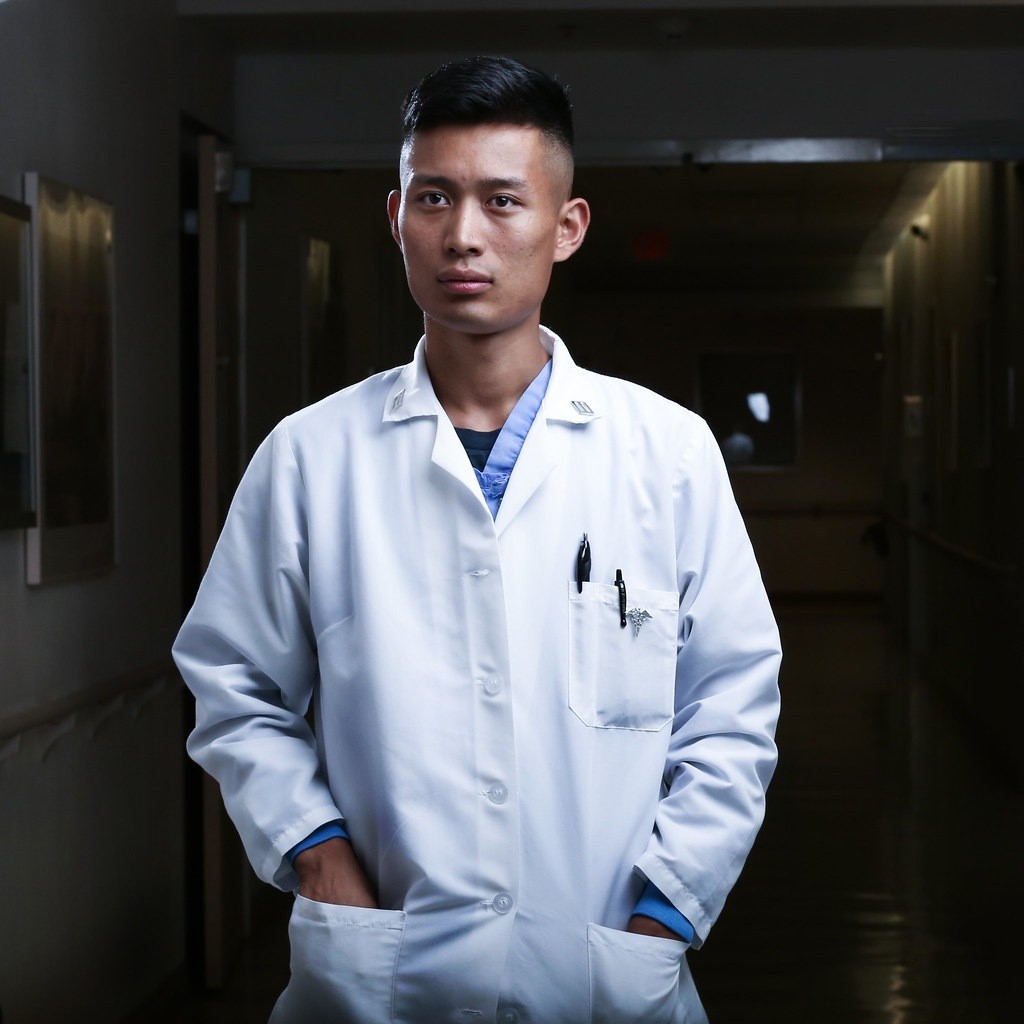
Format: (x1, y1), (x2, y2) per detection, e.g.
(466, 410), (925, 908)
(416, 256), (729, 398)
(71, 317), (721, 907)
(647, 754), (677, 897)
(614, 569), (628, 628)
(576, 533), (592, 594)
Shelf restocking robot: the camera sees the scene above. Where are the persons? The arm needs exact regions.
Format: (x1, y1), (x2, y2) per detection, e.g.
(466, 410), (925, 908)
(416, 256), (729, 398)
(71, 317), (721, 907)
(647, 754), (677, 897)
(172, 56), (783, 1024)
